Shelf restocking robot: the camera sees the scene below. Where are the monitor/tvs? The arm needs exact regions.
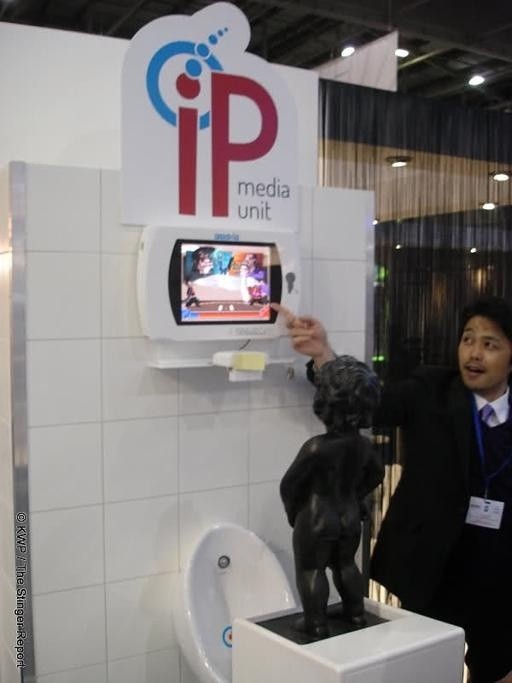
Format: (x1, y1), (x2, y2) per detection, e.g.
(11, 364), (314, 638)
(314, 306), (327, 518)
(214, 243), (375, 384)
(136, 227), (300, 340)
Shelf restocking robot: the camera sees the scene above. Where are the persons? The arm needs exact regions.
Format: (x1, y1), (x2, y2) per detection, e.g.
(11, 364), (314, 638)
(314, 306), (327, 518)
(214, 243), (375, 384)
(279, 352), (386, 636)
(269, 299), (512, 683)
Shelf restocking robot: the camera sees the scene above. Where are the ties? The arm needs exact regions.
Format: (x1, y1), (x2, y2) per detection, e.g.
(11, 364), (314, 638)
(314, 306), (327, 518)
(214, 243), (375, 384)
(479, 403), (495, 422)
